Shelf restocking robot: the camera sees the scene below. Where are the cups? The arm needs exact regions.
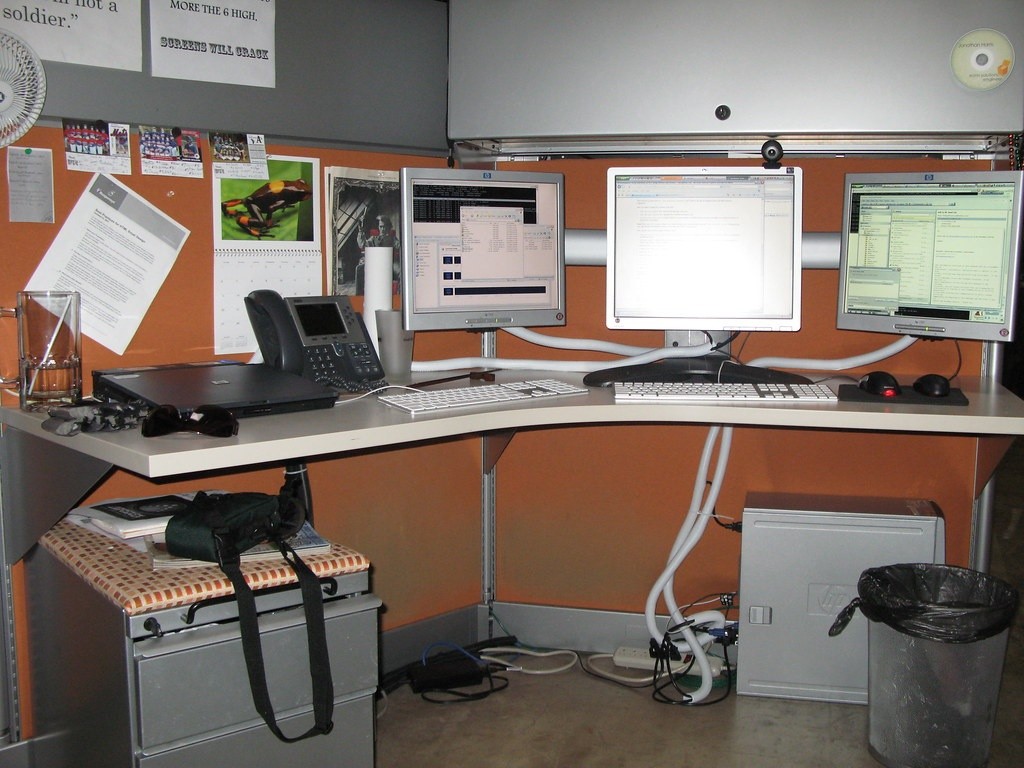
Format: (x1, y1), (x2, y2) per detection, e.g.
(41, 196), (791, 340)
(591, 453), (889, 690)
(375, 309), (414, 386)
(0, 290), (83, 413)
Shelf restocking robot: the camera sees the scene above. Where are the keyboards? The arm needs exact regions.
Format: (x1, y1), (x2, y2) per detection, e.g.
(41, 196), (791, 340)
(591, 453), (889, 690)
(378, 378), (589, 415)
(614, 382), (839, 403)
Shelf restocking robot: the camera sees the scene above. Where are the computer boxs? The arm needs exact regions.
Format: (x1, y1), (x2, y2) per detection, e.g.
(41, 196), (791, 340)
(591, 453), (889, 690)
(736, 492), (946, 704)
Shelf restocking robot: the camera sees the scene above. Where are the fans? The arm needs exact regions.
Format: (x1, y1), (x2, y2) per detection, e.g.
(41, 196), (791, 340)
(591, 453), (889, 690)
(0, 28), (47, 149)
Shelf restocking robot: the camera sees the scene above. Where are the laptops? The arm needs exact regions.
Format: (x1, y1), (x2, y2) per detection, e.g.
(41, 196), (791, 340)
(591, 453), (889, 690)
(100, 361), (339, 418)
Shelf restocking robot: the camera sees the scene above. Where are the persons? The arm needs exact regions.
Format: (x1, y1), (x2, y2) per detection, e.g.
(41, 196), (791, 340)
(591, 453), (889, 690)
(354, 213), (400, 294)
(138, 126), (178, 158)
(213, 132), (246, 161)
(112, 128), (128, 154)
(184, 138), (202, 154)
(63, 123), (109, 155)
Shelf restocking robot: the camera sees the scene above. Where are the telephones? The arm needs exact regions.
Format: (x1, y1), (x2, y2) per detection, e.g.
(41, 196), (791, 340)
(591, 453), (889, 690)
(243, 290), (391, 394)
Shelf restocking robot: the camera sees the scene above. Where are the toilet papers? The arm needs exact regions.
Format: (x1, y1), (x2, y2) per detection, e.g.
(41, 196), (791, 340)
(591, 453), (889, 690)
(361, 244), (395, 368)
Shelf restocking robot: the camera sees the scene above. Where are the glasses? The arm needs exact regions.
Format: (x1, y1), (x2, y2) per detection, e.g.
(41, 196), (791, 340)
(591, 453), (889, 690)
(140, 406), (239, 438)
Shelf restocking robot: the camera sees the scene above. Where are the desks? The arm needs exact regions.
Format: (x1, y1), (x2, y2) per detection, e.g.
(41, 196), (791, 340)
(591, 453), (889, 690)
(0, 370), (1024, 563)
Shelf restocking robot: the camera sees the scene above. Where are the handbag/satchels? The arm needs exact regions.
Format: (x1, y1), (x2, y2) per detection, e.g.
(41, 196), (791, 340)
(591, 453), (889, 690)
(165, 491), (283, 571)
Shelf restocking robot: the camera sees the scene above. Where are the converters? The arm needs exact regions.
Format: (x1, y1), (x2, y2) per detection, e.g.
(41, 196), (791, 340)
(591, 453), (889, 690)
(406, 658), (484, 694)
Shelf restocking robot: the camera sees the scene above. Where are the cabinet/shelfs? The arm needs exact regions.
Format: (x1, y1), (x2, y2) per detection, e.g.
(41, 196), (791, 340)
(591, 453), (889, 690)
(22, 516), (392, 768)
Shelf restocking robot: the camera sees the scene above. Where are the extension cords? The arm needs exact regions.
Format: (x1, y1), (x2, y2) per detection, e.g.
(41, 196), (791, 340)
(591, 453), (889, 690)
(611, 646), (724, 676)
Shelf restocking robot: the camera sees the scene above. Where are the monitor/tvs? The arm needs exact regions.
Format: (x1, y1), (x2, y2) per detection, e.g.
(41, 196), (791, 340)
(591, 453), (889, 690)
(835, 169), (1024, 343)
(582, 166), (803, 387)
(400, 166), (566, 331)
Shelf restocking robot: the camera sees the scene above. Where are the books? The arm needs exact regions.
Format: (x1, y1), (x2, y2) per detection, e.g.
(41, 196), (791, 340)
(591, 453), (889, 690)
(66, 488), (333, 569)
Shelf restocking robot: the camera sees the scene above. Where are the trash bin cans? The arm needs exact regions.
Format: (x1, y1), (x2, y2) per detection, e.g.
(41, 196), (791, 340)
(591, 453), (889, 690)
(862, 562), (1016, 768)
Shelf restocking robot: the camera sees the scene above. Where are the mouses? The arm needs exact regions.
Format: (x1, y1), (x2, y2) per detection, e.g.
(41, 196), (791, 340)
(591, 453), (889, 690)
(913, 374), (951, 397)
(858, 370), (901, 397)
